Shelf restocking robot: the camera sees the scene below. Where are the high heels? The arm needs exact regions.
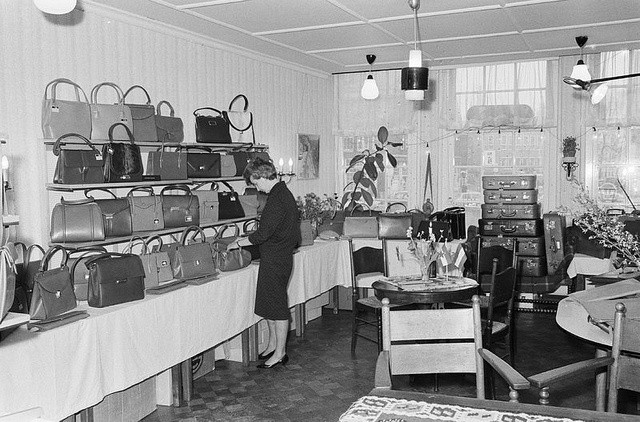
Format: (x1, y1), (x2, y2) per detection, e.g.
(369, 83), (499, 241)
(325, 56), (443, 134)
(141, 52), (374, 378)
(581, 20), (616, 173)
(258, 349), (275, 360)
(256, 353), (289, 369)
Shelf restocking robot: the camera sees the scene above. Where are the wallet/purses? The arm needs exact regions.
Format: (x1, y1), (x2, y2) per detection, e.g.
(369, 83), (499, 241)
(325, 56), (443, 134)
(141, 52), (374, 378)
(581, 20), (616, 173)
(146, 279), (188, 295)
(27, 311), (90, 332)
(185, 272), (220, 286)
(143, 175), (161, 181)
(320, 230), (339, 240)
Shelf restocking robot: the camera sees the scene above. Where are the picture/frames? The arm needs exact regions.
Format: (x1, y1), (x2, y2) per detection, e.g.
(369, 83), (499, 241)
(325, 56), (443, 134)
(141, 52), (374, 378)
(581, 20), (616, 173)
(298, 134), (320, 180)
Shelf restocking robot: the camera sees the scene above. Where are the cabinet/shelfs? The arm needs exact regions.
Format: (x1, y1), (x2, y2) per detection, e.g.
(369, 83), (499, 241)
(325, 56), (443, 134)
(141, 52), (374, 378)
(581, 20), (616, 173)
(42, 138), (263, 249)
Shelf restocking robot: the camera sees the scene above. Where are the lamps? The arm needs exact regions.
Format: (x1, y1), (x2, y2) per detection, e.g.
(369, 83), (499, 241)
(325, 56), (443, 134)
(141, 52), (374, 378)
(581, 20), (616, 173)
(359, 73), (383, 101)
(574, 78), (609, 105)
(399, 48), (432, 104)
(571, 57), (592, 87)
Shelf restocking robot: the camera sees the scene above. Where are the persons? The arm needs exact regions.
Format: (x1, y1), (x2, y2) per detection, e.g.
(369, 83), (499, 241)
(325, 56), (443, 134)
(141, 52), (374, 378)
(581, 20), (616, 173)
(226, 156), (302, 371)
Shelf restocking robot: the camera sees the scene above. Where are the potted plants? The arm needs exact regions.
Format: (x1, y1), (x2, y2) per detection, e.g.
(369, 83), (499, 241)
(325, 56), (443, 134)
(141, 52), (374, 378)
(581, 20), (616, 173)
(560, 135), (582, 157)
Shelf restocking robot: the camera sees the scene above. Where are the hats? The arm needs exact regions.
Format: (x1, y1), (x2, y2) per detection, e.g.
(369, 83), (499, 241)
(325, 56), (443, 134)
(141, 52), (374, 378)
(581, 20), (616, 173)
(243, 157), (265, 186)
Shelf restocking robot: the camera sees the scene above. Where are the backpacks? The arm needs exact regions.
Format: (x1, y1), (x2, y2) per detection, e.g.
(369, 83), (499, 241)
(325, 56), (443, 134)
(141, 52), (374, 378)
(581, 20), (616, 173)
(0, 242), (18, 325)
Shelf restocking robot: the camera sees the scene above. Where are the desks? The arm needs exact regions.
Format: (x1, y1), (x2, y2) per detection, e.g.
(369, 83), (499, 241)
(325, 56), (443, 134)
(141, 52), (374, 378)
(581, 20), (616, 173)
(336, 389), (639, 420)
(372, 274), (482, 303)
(558, 275), (640, 413)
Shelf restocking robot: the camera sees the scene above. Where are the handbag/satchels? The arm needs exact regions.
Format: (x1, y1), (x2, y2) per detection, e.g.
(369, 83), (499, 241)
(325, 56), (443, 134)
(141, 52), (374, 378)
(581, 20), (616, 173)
(212, 223), (251, 271)
(50, 195), (106, 242)
(211, 181), (245, 220)
(174, 225), (216, 279)
(249, 148), (273, 164)
(240, 218), (263, 261)
(147, 144), (187, 180)
(417, 211), (454, 242)
(317, 199), (343, 237)
(192, 107), (222, 143)
(84, 252), (146, 308)
(123, 235), (173, 289)
(407, 209), (431, 238)
(159, 184), (200, 227)
(238, 194), (260, 217)
(29, 245), (76, 319)
(231, 143), (253, 176)
(121, 185), (164, 231)
(343, 203), (379, 238)
(444, 206), (466, 240)
(53, 132), (104, 184)
(11, 242), (49, 314)
(89, 82), (134, 140)
(186, 145), (220, 178)
(212, 149), (237, 177)
(66, 245), (108, 300)
(211, 239), (251, 272)
(222, 94), (255, 144)
(379, 201), (413, 241)
(190, 181), (219, 224)
(122, 85), (154, 143)
(300, 219), (314, 245)
(84, 188), (132, 236)
(153, 100), (184, 143)
(102, 123), (144, 182)
(41, 79), (91, 141)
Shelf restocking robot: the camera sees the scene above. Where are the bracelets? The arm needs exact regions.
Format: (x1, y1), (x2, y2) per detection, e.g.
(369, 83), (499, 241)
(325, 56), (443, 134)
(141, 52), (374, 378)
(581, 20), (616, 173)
(236, 240), (240, 246)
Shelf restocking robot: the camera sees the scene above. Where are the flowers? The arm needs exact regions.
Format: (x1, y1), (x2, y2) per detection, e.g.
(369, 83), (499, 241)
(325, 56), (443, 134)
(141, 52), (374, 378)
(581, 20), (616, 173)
(296, 193), (339, 223)
(405, 225), (448, 269)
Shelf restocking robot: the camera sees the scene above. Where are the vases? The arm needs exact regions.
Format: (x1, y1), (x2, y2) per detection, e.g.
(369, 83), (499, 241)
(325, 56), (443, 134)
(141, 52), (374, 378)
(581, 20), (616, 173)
(420, 267), (431, 282)
(298, 220), (314, 247)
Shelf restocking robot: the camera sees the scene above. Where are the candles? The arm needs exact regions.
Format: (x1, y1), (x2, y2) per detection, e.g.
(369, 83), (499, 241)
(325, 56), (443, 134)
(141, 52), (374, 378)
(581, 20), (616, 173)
(288, 158), (295, 174)
(278, 158), (284, 175)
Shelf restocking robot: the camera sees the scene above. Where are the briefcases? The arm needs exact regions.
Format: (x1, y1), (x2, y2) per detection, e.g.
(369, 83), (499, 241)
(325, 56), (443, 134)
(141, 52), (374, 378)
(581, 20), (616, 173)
(516, 257), (542, 277)
(483, 190), (539, 204)
(480, 275), (561, 293)
(480, 204), (541, 220)
(481, 237), (542, 256)
(478, 219), (542, 237)
(484, 292), (538, 312)
(542, 210), (565, 276)
(603, 209), (640, 268)
(572, 213), (611, 260)
(482, 176), (536, 190)
(533, 286), (569, 313)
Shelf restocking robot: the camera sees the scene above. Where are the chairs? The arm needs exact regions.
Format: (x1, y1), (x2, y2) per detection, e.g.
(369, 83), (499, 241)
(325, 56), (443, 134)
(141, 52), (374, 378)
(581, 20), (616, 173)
(346, 235), (390, 356)
(527, 303), (640, 415)
(374, 297), (530, 402)
(473, 231), (517, 309)
(479, 256), (520, 361)
